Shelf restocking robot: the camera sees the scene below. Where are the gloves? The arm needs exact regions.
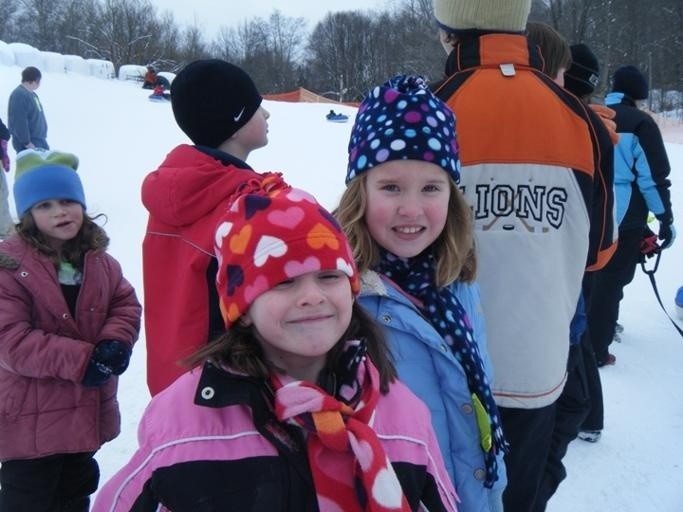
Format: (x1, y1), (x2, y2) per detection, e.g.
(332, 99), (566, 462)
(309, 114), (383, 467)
(657, 216), (677, 250)
(637, 223), (659, 259)
(587, 102), (619, 144)
(99, 339), (131, 376)
(82, 359), (113, 388)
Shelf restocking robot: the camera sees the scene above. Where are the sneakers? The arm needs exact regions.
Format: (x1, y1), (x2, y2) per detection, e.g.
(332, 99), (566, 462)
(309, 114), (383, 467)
(578, 424), (602, 443)
(612, 334), (622, 343)
(596, 352), (616, 368)
(615, 322), (624, 333)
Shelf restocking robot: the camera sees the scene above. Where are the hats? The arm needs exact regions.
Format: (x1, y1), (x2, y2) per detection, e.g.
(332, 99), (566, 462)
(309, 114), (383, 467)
(345, 73), (461, 186)
(611, 64), (649, 98)
(563, 43), (599, 89)
(207, 171), (361, 331)
(12, 149), (86, 221)
(170, 58), (264, 149)
(432, 0), (532, 37)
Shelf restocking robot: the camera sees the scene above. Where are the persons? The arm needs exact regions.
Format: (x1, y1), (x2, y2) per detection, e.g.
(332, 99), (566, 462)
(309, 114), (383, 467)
(7, 67), (52, 158)
(144, 67), (155, 85)
(605, 63), (675, 331)
(566, 43), (616, 364)
(526, 20), (617, 443)
(0, 118), (14, 239)
(154, 83), (163, 95)
(326, 73), (510, 511)
(85, 172), (462, 511)
(0, 147), (142, 512)
(141, 59), (273, 397)
(422, 0), (621, 511)
